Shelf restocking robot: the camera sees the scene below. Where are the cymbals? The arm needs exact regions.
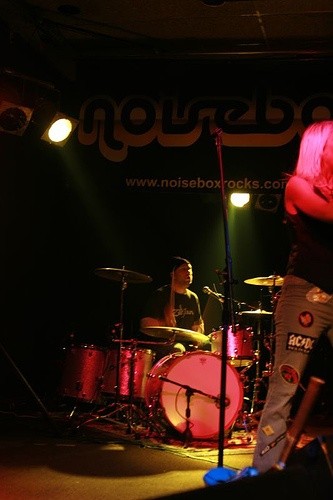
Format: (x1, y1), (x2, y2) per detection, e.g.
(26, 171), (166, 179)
(95, 267), (153, 284)
(138, 326), (211, 341)
(236, 309), (274, 318)
(243, 276), (284, 286)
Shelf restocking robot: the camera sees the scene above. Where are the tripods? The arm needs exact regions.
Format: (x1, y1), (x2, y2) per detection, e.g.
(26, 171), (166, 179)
(88, 275), (163, 437)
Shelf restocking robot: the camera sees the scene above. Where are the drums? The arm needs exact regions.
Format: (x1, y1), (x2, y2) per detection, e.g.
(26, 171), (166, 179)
(141, 349), (243, 440)
(55, 342), (109, 406)
(97, 343), (157, 400)
(207, 323), (255, 368)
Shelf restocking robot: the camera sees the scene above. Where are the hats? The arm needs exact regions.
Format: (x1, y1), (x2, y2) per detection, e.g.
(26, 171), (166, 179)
(164, 257), (188, 272)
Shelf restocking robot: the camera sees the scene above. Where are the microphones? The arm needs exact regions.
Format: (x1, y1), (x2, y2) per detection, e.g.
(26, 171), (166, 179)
(220, 395), (230, 406)
(202, 286), (224, 303)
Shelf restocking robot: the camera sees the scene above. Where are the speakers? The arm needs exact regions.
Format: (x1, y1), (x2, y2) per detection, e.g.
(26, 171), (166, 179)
(287, 433), (333, 500)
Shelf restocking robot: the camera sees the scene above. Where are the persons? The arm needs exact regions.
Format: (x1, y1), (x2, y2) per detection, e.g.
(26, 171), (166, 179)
(252, 120), (333, 472)
(137, 256), (205, 360)
